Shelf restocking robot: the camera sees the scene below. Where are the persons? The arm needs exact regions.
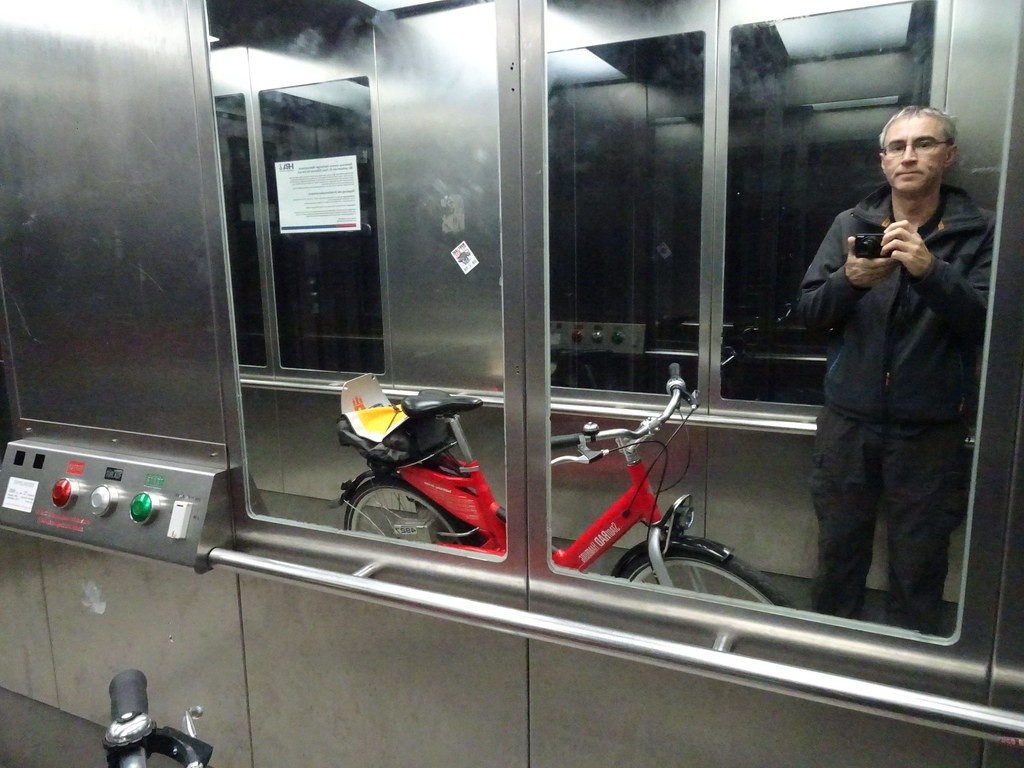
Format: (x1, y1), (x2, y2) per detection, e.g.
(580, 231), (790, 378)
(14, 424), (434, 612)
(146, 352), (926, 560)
(795, 102), (997, 636)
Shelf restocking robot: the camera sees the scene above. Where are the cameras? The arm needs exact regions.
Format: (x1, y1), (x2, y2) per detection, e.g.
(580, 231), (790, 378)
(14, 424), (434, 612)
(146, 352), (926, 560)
(855, 234), (893, 259)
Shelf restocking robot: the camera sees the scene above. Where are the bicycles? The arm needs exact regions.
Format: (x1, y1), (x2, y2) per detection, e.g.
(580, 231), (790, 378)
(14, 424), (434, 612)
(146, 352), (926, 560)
(328, 361), (793, 615)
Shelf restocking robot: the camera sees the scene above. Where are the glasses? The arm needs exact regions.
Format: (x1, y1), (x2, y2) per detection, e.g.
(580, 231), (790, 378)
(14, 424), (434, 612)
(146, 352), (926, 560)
(880, 139), (952, 157)
